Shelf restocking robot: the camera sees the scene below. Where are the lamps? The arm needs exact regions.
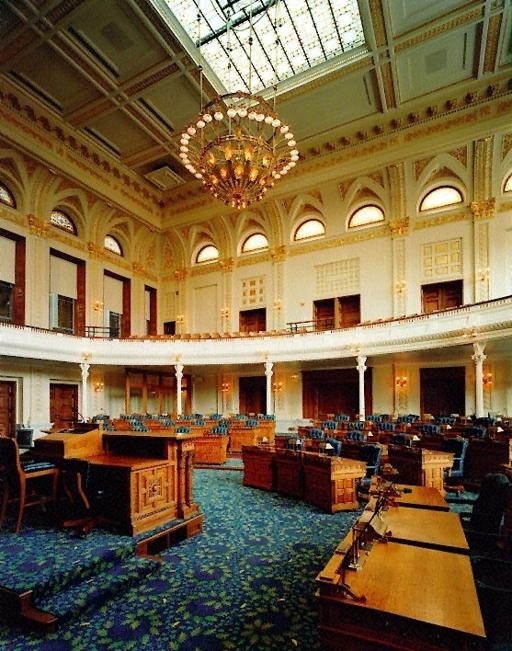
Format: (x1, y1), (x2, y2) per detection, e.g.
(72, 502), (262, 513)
(178, 0), (299, 209)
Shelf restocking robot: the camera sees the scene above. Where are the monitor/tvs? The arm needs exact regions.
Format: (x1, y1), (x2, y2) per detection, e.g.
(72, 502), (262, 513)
(17, 427), (48, 449)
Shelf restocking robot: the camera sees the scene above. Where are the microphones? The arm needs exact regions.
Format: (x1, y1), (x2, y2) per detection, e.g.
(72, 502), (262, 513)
(69, 405), (86, 422)
